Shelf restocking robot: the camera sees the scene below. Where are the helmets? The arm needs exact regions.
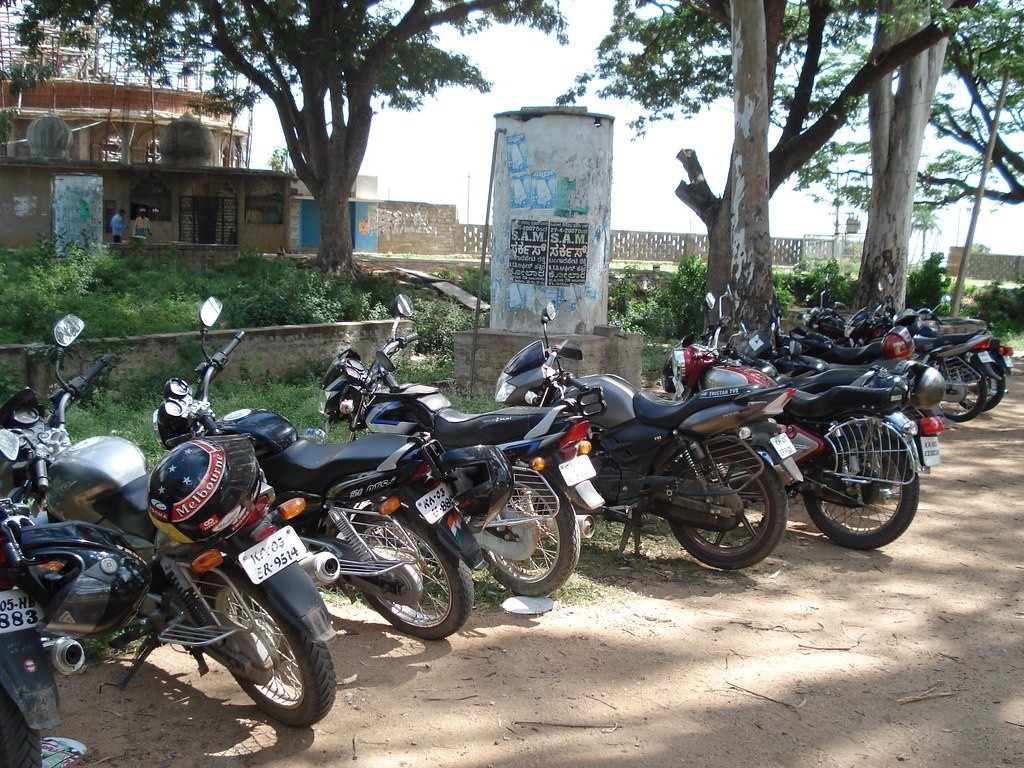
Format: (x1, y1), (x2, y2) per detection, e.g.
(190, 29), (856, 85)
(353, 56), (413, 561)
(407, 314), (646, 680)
(844, 307), (876, 339)
(144, 434), (264, 546)
(895, 360), (946, 410)
(439, 444), (516, 535)
(19, 521), (152, 641)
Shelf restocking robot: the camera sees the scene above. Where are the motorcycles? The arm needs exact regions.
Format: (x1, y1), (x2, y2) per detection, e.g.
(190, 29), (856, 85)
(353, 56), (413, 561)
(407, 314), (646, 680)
(0, 497), (152, 768)
(493, 301), (802, 573)
(0, 312), (342, 729)
(152, 294), (516, 644)
(317, 293), (605, 598)
(660, 287), (1016, 552)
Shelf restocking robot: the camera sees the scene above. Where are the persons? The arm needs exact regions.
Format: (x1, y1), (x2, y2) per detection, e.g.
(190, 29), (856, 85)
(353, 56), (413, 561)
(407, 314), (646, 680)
(110, 209), (127, 243)
(132, 207), (152, 237)
